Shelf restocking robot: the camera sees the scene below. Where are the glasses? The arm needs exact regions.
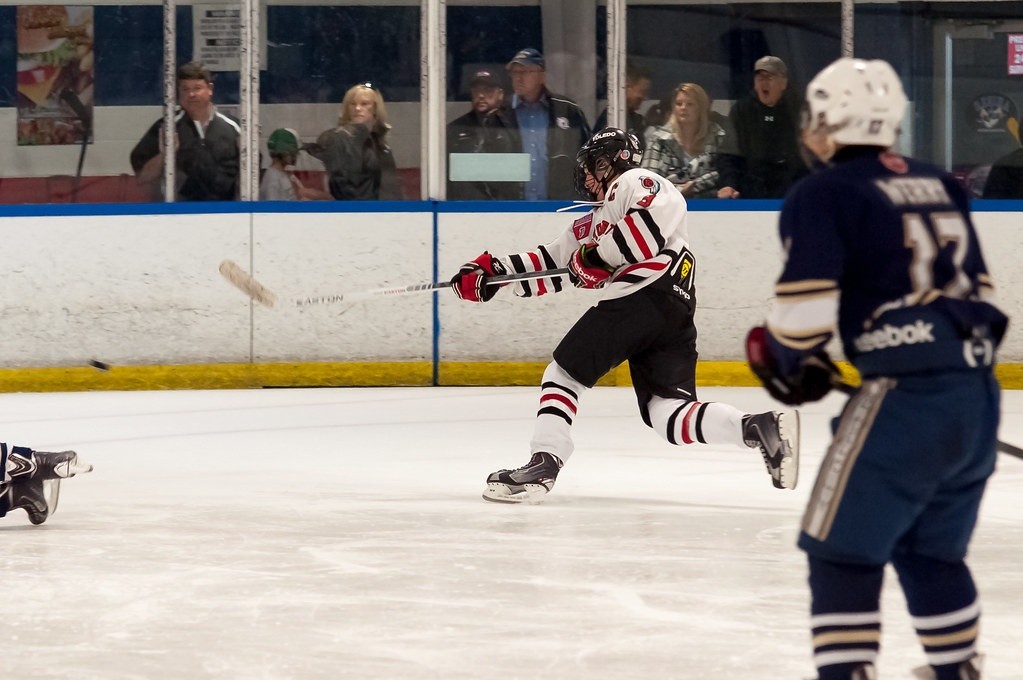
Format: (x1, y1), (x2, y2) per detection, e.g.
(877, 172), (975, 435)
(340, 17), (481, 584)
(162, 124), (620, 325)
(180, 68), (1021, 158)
(470, 88), (496, 97)
(756, 75), (777, 84)
(508, 71), (531, 78)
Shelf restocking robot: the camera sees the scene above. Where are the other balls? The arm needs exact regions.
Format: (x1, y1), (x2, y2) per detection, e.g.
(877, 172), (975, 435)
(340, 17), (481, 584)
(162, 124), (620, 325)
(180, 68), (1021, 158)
(89, 359), (110, 371)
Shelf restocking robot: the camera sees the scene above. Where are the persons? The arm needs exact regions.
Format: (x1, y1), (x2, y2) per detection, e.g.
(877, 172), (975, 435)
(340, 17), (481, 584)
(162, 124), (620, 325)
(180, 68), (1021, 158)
(450, 127), (786, 493)
(717, 56), (810, 198)
(592, 71), (726, 198)
(472, 46), (591, 201)
(130, 61), (263, 202)
(0, 443), (76, 524)
(744, 54), (1010, 680)
(295, 83), (404, 201)
(447, 70), (504, 200)
(259, 126), (312, 200)
(982, 117), (1023, 202)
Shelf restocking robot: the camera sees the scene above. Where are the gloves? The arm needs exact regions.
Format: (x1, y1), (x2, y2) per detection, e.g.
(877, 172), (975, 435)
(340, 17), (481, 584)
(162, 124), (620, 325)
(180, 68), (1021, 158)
(449, 249), (512, 304)
(743, 320), (845, 407)
(565, 242), (614, 290)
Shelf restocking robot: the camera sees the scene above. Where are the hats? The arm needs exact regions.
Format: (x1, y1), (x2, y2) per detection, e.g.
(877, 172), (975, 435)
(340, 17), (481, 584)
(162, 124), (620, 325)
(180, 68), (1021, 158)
(752, 55), (790, 79)
(268, 127), (304, 159)
(468, 68), (505, 89)
(505, 48), (545, 73)
(177, 59), (212, 80)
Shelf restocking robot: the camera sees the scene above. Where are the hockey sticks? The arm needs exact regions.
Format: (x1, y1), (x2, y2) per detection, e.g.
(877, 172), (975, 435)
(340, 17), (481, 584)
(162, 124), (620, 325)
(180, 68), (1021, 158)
(827, 378), (1022, 461)
(220, 259), (569, 313)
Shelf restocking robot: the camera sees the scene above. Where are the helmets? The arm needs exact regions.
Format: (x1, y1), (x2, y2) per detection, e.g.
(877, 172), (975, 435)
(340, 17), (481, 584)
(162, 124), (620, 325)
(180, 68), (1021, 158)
(573, 127), (644, 200)
(804, 55), (907, 146)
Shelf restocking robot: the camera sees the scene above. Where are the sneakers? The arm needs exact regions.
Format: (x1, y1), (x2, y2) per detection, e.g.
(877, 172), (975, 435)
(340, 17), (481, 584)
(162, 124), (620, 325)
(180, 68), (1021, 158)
(30, 448), (95, 480)
(482, 452), (565, 504)
(9, 478), (61, 525)
(742, 409), (801, 491)
